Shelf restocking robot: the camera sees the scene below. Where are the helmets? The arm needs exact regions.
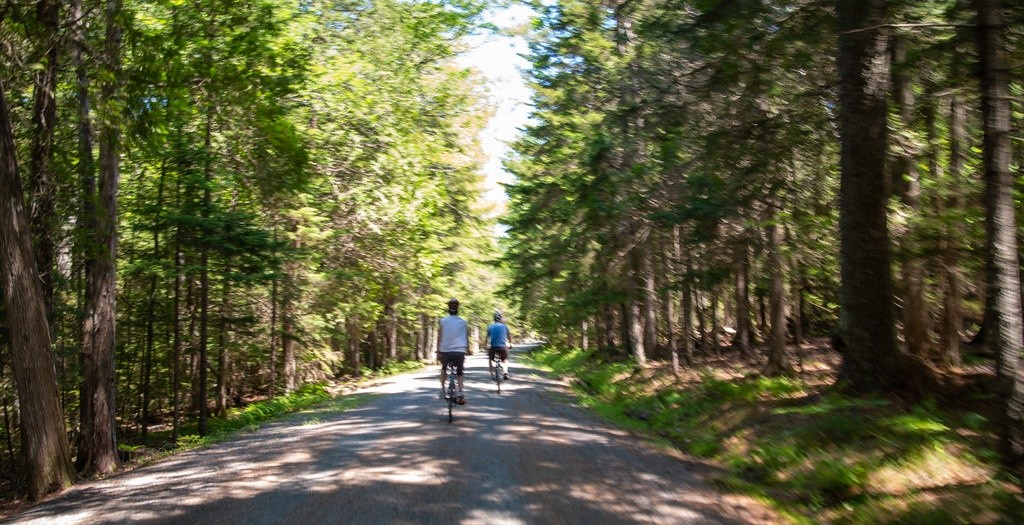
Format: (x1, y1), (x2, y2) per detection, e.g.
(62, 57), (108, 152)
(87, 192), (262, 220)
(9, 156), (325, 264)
(494, 313), (501, 321)
(449, 299), (459, 308)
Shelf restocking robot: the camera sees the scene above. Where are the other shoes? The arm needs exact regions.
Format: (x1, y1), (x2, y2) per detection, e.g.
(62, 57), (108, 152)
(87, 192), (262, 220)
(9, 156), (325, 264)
(504, 373), (509, 380)
(459, 398), (465, 404)
(491, 378), (497, 384)
(440, 392), (446, 399)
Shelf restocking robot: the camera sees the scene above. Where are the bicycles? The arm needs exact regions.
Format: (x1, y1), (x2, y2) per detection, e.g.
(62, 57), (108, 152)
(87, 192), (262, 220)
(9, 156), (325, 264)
(434, 348), (472, 423)
(484, 345), (511, 395)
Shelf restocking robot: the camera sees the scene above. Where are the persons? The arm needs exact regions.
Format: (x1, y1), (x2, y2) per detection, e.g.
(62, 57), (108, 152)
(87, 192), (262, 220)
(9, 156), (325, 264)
(483, 313), (511, 381)
(437, 299), (471, 404)
